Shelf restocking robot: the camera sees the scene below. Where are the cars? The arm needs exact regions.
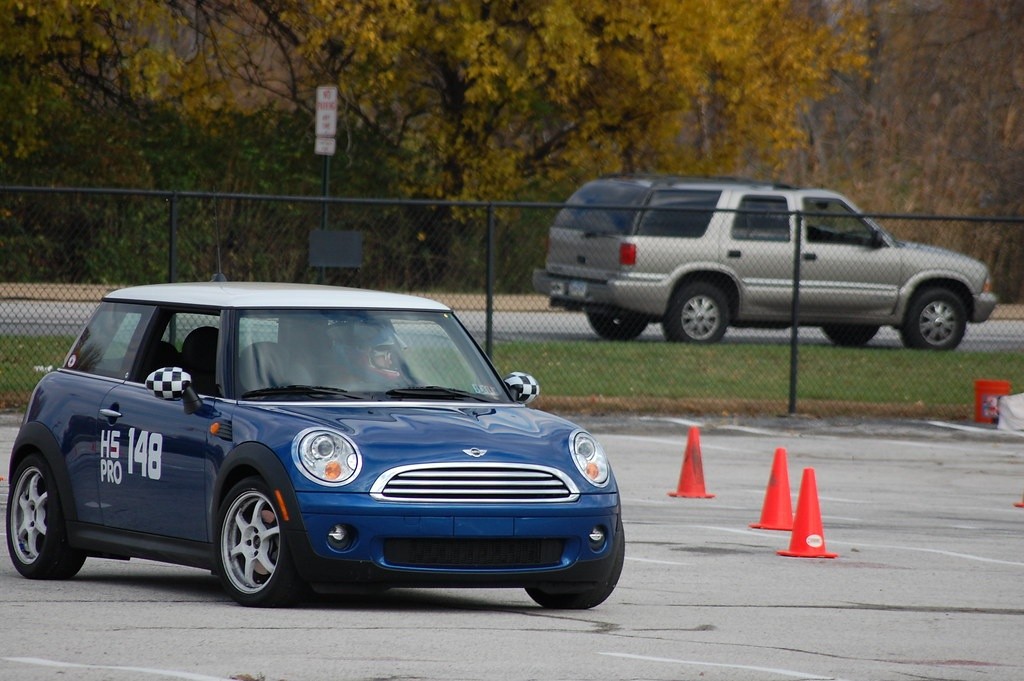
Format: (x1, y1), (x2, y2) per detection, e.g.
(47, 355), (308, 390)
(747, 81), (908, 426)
(5, 282), (627, 610)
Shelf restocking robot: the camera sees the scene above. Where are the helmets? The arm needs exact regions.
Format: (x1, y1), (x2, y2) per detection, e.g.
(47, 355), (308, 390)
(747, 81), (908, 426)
(340, 319), (407, 387)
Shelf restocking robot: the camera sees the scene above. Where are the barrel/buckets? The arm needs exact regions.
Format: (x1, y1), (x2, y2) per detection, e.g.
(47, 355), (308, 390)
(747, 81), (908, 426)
(974, 380), (1009, 422)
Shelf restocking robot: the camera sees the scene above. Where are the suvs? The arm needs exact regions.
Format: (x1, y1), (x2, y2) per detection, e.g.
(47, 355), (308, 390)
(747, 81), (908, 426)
(533, 176), (999, 352)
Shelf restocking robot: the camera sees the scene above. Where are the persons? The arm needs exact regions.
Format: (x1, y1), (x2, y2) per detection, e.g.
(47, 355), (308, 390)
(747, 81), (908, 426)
(326, 319), (409, 392)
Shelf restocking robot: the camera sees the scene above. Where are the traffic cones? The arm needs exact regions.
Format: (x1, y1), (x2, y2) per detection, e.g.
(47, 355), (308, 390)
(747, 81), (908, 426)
(747, 446), (794, 531)
(779, 466), (839, 559)
(665, 424), (717, 499)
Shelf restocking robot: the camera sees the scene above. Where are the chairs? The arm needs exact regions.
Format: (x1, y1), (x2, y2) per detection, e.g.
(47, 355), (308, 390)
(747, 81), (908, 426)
(139, 326), (313, 398)
